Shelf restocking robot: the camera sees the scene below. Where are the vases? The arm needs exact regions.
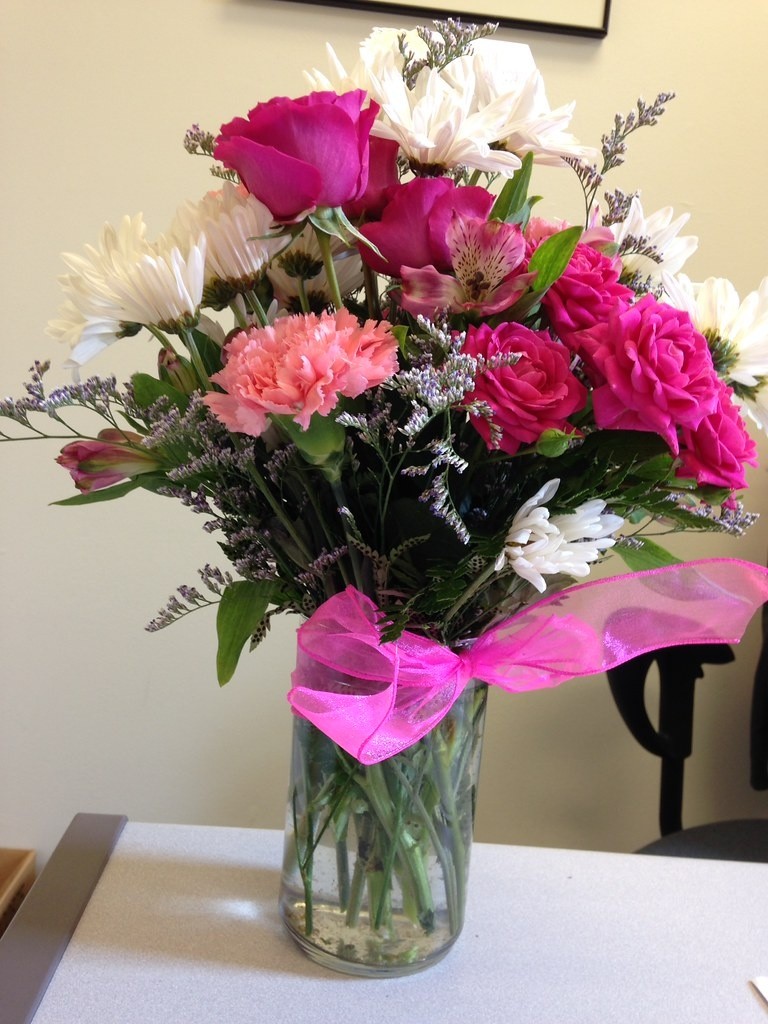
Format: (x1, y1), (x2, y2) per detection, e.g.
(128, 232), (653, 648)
(276, 632), (489, 980)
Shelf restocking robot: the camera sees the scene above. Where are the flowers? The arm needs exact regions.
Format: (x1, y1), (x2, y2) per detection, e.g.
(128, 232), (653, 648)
(0, 12), (768, 934)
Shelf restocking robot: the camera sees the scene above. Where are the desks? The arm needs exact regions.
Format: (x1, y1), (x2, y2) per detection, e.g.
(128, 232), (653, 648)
(0, 813), (768, 1024)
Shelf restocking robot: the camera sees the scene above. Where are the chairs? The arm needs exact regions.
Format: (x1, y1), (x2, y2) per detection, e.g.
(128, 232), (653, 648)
(603, 599), (768, 868)
